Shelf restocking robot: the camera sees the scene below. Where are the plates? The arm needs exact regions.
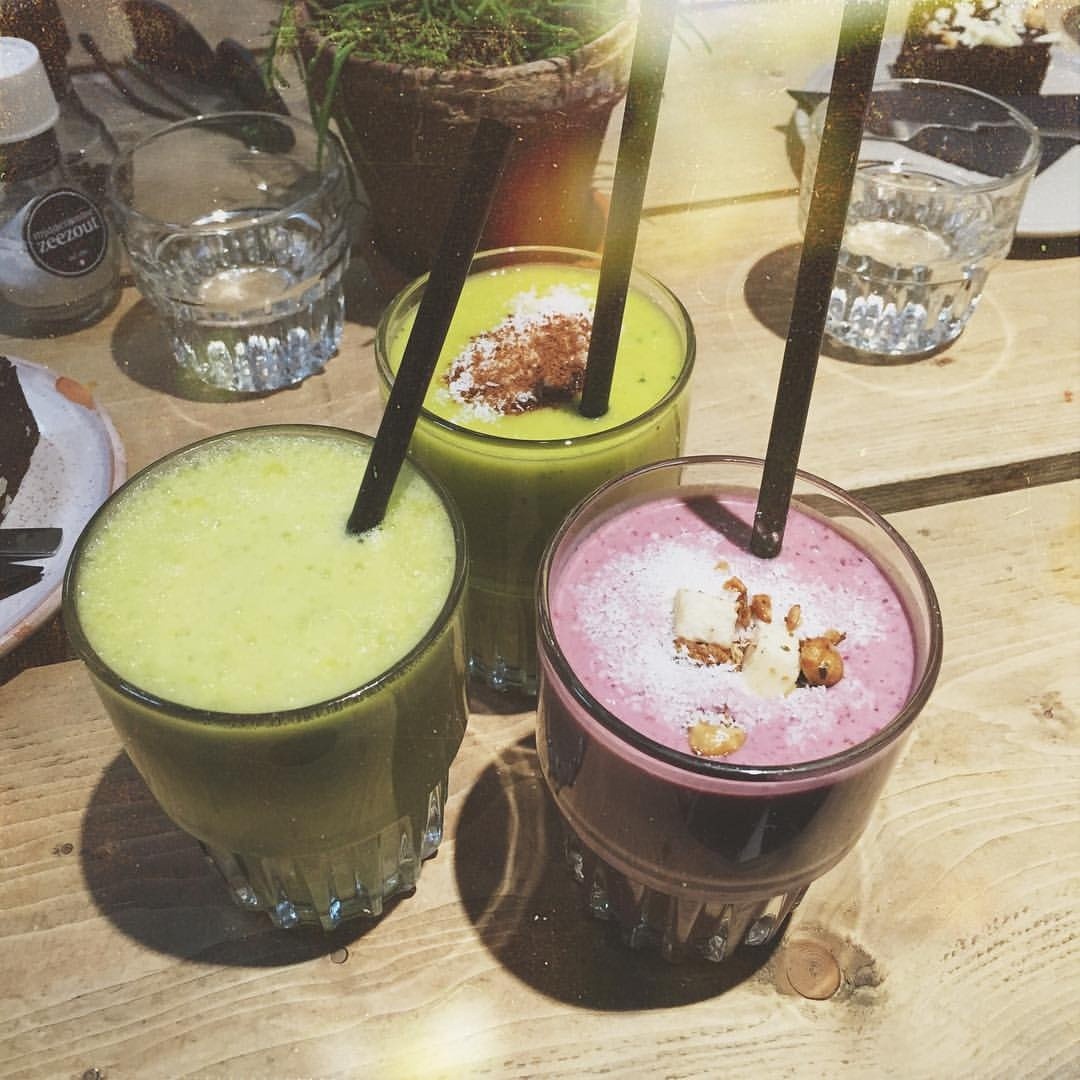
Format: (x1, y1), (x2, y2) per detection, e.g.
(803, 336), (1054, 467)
(796, 38), (1078, 237)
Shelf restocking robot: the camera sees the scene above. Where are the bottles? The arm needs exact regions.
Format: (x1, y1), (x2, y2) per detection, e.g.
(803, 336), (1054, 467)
(0, 34), (117, 341)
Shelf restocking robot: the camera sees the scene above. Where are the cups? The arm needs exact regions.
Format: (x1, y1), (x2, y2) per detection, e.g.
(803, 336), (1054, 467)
(534, 454), (943, 963)
(60, 424), (466, 931)
(372, 244), (696, 700)
(106, 111), (345, 394)
(801, 78), (1043, 350)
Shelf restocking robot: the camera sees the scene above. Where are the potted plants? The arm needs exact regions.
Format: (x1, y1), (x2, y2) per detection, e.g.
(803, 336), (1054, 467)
(253, 0), (644, 269)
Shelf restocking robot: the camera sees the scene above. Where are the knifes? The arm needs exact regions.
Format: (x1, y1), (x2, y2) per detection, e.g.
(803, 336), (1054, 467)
(788, 87), (1078, 146)
